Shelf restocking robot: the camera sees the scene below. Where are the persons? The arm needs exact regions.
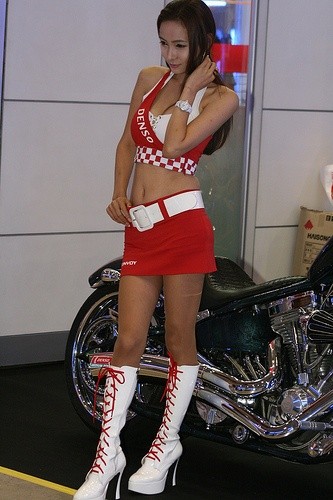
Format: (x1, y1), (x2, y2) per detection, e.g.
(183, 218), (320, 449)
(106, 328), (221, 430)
(71, 1), (240, 500)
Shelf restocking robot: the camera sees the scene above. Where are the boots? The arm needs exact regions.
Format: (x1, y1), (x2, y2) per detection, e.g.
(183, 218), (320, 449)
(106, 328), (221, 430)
(130, 361), (201, 494)
(73, 365), (139, 500)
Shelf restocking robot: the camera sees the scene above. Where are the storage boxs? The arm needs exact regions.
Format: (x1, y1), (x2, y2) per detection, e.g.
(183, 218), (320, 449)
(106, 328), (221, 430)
(292, 206), (333, 276)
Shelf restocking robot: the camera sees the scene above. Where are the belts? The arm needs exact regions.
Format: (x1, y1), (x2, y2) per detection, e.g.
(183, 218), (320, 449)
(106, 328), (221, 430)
(126, 189), (206, 232)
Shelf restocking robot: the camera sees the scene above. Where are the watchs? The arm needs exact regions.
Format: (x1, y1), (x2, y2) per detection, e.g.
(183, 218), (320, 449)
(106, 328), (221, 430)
(174, 100), (193, 114)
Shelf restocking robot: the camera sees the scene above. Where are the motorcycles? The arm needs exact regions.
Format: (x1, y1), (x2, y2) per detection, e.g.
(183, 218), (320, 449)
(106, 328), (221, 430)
(67, 230), (333, 466)
